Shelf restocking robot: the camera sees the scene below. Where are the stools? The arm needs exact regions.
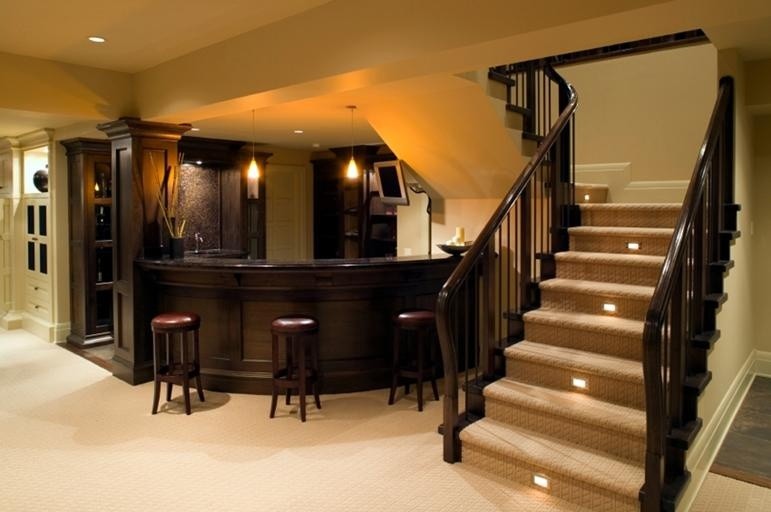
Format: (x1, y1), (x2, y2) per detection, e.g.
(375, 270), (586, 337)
(151, 311), (205, 414)
(270, 317), (322, 422)
(388, 311), (439, 412)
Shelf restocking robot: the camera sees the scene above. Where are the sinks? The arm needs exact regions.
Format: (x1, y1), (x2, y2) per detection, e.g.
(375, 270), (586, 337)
(189, 250), (224, 255)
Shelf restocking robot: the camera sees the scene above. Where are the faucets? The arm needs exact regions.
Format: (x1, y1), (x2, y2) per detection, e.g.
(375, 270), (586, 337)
(194, 231), (205, 253)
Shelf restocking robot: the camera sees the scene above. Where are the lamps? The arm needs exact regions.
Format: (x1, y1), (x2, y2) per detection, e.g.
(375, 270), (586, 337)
(247, 109), (259, 179)
(346, 106), (359, 178)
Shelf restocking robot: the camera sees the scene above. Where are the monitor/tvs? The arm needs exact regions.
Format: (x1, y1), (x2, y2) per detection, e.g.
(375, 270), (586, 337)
(374, 160), (409, 205)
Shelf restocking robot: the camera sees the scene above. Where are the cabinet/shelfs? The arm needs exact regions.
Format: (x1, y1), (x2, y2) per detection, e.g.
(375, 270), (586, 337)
(59, 137), (112, 350)
(21, 198), (53, 324)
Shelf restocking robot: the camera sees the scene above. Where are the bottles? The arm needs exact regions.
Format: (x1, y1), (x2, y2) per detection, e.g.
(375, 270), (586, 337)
(96, 255), (103, 282)
(97, 206), (106, 226)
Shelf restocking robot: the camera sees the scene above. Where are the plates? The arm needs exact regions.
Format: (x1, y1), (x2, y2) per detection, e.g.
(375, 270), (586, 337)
(436, 242), (474, 255)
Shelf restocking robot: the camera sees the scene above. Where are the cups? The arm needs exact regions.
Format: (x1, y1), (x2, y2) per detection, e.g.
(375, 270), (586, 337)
(169, 238), (184, 259)
